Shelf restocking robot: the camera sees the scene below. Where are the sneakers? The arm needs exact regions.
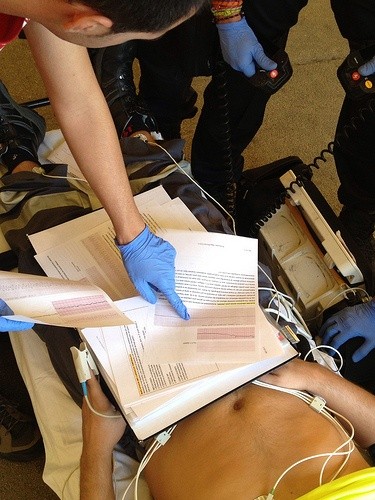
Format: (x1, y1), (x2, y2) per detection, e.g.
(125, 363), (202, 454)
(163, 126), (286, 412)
(0, 395), (44, 462)
(92, 38), (158, 138)
(0, 78), (47, 168)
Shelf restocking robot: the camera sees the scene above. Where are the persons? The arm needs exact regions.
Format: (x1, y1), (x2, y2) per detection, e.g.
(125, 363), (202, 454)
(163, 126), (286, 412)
(139, 0), (375, 362)
(0, 0), (204, 461)
(0, 41), (375, 500)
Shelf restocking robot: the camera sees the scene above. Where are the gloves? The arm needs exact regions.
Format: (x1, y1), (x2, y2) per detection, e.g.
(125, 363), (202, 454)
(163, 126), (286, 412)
(316, 298), (374, 365)
(114, 223), (193, 322)
(1, 299), (34, 331)
(215, 15), (278, 77)
